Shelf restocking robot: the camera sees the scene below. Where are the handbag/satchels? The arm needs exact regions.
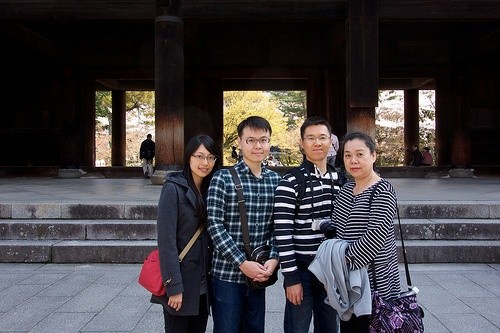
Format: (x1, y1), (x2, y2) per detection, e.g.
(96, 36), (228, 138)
(369, 286), (424, 333)
(246, 245), (280, 290)
(139, 248), (167, 297)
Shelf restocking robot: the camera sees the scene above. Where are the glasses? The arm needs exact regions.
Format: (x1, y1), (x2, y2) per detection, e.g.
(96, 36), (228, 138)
(240, 137), (271, 145)
(191, 155), (217, 162)
(304, 136), (330, 142)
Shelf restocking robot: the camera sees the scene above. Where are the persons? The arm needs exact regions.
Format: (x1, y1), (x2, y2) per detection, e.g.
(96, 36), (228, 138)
(150, 134), (221, 333)
(231, 146), (282, 166)
(207, 116), (282, 333)
(273, 117), (347, 333)
(320, 132), (402, 333)
(406, 144), (433, 167)
(327, 132), (339, 168)
(139, 134), (155, 179)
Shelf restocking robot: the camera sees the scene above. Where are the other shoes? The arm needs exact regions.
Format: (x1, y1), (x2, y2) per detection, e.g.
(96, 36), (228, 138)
(147, 176), (150, 179)
(144, 172), (148, 179)
